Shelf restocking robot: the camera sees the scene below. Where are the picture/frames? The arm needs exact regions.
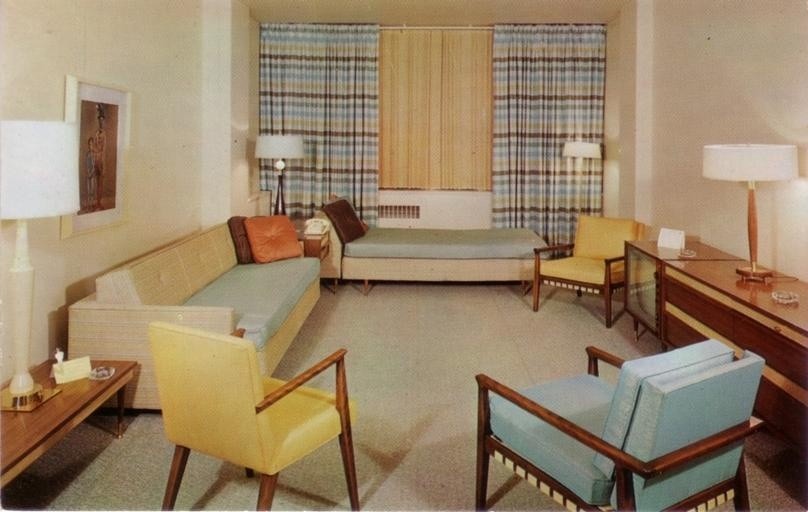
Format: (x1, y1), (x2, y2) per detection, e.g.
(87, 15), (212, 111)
(61, 74), (132, 225)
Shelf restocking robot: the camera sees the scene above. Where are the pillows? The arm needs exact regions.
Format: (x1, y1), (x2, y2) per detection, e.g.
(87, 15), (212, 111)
(227, 214), (255, 265)
(321, 194), (369, 242)
(243, 212), (302, 265)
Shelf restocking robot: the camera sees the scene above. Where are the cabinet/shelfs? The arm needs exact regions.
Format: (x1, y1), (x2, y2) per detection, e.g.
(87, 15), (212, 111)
(621, 236), (744, 341)
(661, 257), (808, 451)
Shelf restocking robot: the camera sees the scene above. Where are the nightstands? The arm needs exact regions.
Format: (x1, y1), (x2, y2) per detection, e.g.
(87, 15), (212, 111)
(288, 219), (334, 296)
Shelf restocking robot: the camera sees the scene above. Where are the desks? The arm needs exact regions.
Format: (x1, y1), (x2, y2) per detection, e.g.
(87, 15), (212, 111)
(1, 356), (138, 501)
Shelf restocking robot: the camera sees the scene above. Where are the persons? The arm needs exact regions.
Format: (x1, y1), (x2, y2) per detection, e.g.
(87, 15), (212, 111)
(92, 111), (106, 210)
(83, 137), (97, 212)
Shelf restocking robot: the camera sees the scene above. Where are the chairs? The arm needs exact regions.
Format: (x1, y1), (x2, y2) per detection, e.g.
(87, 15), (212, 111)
(145, 319), (363, 509)
(470, 336), (768, 509)
(530, 214), (644, 327)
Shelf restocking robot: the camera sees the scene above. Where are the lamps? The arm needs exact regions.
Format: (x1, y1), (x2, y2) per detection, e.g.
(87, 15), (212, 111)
(253, 134), (306, 217)
(701, 139), (802, 280)
(562, 141), (604, 216)
(1, 120), (81, 412)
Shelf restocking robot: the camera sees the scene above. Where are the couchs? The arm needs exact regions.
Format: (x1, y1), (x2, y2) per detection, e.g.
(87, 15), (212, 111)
(65, 226), (322, 418)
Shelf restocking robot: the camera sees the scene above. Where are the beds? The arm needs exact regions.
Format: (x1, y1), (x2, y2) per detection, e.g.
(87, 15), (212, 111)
(342, 226), (554, 296)
